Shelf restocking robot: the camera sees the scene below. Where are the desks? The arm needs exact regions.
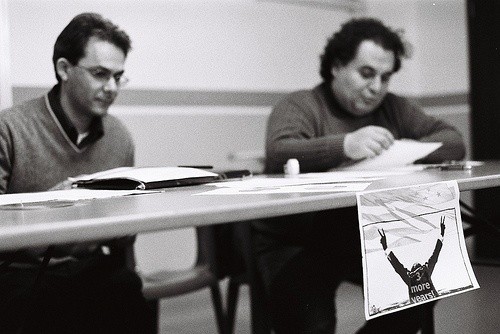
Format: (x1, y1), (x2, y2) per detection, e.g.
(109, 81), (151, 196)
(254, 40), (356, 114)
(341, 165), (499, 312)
(0, 161), (500, 334)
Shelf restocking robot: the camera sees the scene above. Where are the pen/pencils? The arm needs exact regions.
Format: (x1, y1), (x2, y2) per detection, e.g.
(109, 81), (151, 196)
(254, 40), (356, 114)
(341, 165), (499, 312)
(439, 164), (470, 171)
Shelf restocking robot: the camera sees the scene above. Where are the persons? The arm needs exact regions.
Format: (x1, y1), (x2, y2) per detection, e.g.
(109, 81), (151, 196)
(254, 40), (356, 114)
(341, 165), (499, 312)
(249, 16), (465, 334)
(1, 12), (148, 334)
(377, 216), (445, 304)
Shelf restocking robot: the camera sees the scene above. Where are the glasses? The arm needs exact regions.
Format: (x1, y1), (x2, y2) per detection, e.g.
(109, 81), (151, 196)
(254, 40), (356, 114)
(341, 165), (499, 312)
(70, 60), (128, 88)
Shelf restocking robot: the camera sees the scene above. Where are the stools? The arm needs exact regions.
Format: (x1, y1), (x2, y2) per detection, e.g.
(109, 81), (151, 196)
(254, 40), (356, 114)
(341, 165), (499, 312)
(133, 264), (249, 334)
